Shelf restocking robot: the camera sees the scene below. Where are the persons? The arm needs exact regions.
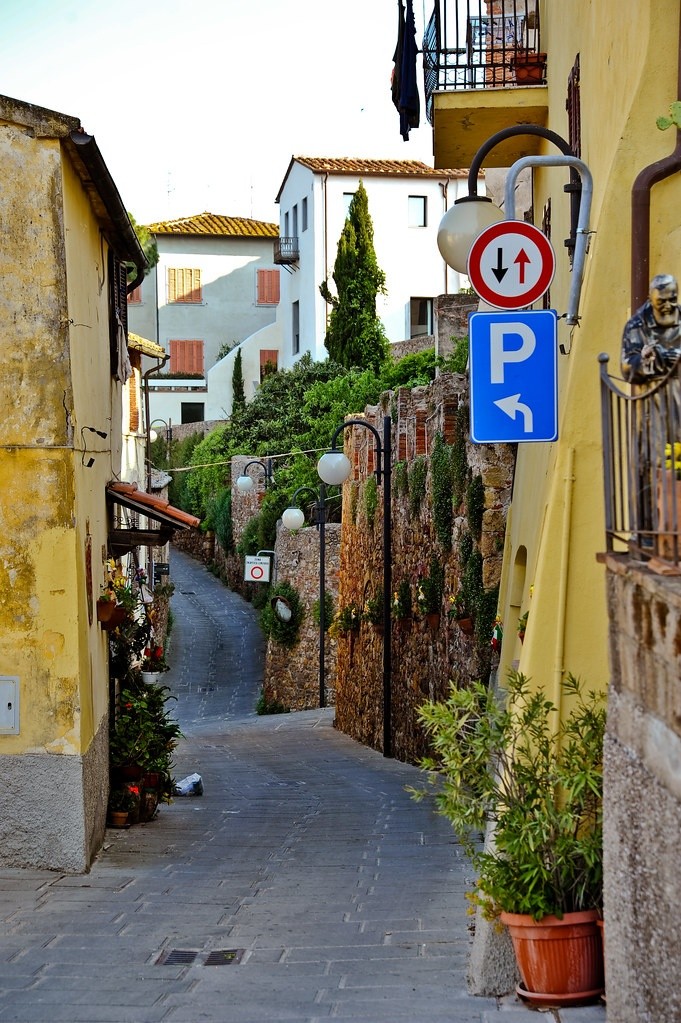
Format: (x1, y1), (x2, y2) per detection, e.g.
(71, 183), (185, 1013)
(621, 272), (681, 544)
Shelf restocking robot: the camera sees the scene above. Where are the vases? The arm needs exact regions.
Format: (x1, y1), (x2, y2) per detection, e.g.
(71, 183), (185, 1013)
(458, 618), (472, 635)
(141, 671), (159, 685)
(427, 615), (439, 630)
(373, 624), (381, 632)
(142, 789), (158, 821)
(142, 772), (158, 787)
(110, 811), (130, 829)
(119, 765), (141, 781)
(123, 782), (140, 822)
(654, 474), (681, 557)
(96, 601), (115, 622)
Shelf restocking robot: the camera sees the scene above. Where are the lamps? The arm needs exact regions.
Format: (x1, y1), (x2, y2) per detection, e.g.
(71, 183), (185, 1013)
(81, 425), (108, 469)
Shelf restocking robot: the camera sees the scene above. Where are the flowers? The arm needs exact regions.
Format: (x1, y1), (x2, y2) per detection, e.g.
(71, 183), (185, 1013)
(97, 589), (116, 601)
(362, 600), (380, 624)
(660, 438), (681, 471)
(448, 593), (467, 620)
(417, 594), (432, 614)
(143, 644), (164, 670)
(114, 683), (179, 804)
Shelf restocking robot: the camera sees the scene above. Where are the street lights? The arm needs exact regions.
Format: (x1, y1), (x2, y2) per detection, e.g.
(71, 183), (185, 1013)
(236, 459), (275, 595)
(145, 416), (173, 477)
(315, 417), (393, 758)
(281, 486), (333, 707)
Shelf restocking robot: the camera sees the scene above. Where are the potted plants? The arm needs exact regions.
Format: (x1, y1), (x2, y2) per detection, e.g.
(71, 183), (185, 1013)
(406, 665), (606, 1006)
(480, 19), (547, 87)
